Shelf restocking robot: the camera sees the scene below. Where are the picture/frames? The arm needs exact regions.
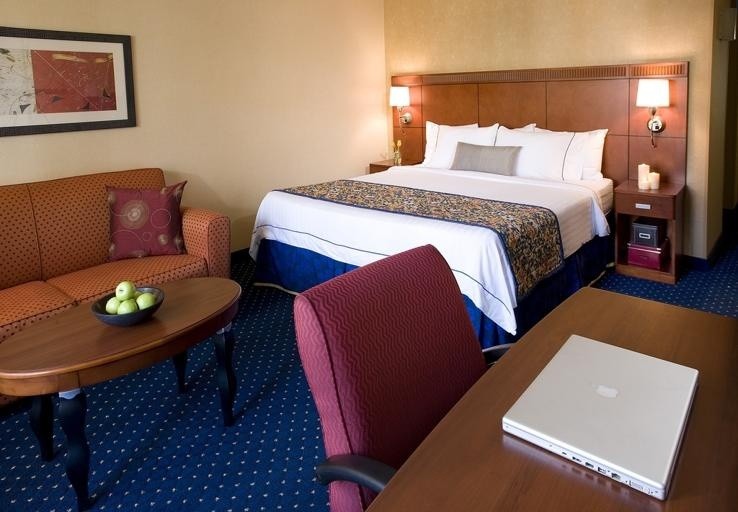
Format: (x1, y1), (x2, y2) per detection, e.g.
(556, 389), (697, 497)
(0, 25), (138, 138)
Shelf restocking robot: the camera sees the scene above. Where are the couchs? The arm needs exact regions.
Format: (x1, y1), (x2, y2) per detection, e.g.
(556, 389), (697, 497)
(2, 167), (233, 421)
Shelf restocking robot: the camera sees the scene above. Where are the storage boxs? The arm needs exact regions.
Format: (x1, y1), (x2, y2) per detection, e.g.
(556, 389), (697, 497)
(625, 242), (670, 271)
(631, 220), (659, 248)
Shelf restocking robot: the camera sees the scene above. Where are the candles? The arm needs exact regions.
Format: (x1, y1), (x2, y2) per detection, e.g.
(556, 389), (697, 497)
(637, 163), (662, 190)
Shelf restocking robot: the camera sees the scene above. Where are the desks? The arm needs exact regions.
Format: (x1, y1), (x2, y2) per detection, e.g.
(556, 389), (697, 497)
(359, 286), (737, 511)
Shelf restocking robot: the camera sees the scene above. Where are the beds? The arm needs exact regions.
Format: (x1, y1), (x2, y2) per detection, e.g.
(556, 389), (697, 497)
(249, 160), (614, 352)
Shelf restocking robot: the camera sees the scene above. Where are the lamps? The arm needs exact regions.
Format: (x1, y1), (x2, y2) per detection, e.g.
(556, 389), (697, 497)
(633, 76), (671, 150)
(390, 84), (414, 123)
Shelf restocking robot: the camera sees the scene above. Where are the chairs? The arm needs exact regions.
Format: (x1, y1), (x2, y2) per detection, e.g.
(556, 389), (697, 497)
(287, 241), (519, 510)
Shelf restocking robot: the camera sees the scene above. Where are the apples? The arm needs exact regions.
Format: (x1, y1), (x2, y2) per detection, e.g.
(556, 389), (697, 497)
(105, 281), (158, 315)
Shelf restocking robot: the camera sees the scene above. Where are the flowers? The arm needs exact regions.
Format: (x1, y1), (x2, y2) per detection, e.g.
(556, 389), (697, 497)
(392, 139), (403, 165)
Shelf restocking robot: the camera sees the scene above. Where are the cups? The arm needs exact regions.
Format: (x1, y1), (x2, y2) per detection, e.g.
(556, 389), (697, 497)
(637, 164), (660, 189)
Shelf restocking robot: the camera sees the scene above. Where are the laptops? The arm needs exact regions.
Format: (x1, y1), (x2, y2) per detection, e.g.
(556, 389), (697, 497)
(501, 334), (700, 499)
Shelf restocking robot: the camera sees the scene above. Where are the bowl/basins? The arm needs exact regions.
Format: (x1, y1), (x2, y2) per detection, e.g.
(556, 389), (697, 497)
(90, 287), (164, 327)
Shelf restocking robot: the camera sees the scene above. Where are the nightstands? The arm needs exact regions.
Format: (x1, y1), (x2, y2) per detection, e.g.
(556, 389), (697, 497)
(610, 175), (685, 286)
(369, 157), (420, 173)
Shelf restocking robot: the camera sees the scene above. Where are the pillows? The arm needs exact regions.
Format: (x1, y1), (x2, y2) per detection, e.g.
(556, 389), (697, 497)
(104, 177), (192, 259)
(422, 119), (611, 189)
